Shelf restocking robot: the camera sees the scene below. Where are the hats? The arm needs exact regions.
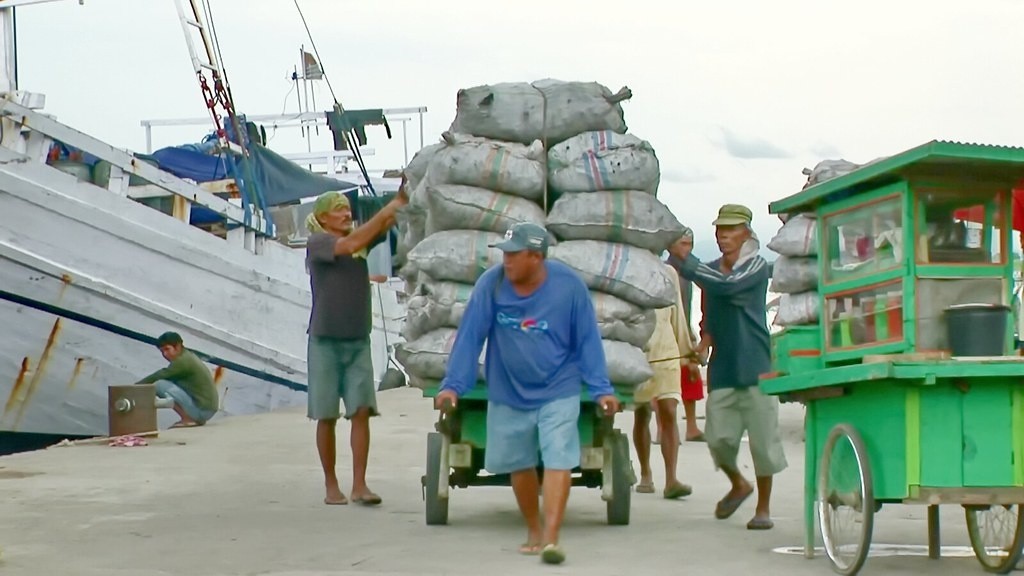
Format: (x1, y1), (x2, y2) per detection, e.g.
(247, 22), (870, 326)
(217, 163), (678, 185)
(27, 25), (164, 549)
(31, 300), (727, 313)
(488, 222), (548, 256)
(712, 204), (753, 227)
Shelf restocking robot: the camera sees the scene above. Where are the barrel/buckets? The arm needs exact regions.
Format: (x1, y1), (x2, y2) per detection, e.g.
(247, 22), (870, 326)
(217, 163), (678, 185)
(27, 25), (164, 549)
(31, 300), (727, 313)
(941, 302), (1011, 358)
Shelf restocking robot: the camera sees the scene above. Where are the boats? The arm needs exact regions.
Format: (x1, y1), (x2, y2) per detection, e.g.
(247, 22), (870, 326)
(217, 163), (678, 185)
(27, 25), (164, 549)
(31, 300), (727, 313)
(1, 0), (431, 437)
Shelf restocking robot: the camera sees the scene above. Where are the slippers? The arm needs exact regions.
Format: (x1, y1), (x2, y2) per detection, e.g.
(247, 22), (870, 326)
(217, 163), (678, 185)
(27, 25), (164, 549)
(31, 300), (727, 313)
(686, 431), (707, 442)
(636, 482), (655, 493)
(747, 518), (774, 529)
(521, 542), (541, 555)
(716, 487), (753, 519)
(541, 542), (564, 564)
(172, 421), (203, 427)
(664, 481), (691, 499)
(654, 440), (683, 445)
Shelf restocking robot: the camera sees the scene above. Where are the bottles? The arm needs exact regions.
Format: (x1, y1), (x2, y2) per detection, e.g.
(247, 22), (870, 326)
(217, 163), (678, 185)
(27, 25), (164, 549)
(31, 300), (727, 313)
(831, 290), (904, 349)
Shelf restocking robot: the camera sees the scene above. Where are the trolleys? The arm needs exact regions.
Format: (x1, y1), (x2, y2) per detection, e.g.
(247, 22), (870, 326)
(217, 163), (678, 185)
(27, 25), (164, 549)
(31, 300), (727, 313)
(755, 139), (1023, 576)
(419, 380), (651, 527)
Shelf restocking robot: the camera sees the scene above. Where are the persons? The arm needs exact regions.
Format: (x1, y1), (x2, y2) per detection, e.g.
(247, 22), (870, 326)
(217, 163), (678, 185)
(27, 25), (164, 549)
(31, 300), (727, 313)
(632, 204), (789, 529)
(436, 223), (619, 565)
(133, 332), (219, 429)
(306, 177), (407, 505)
